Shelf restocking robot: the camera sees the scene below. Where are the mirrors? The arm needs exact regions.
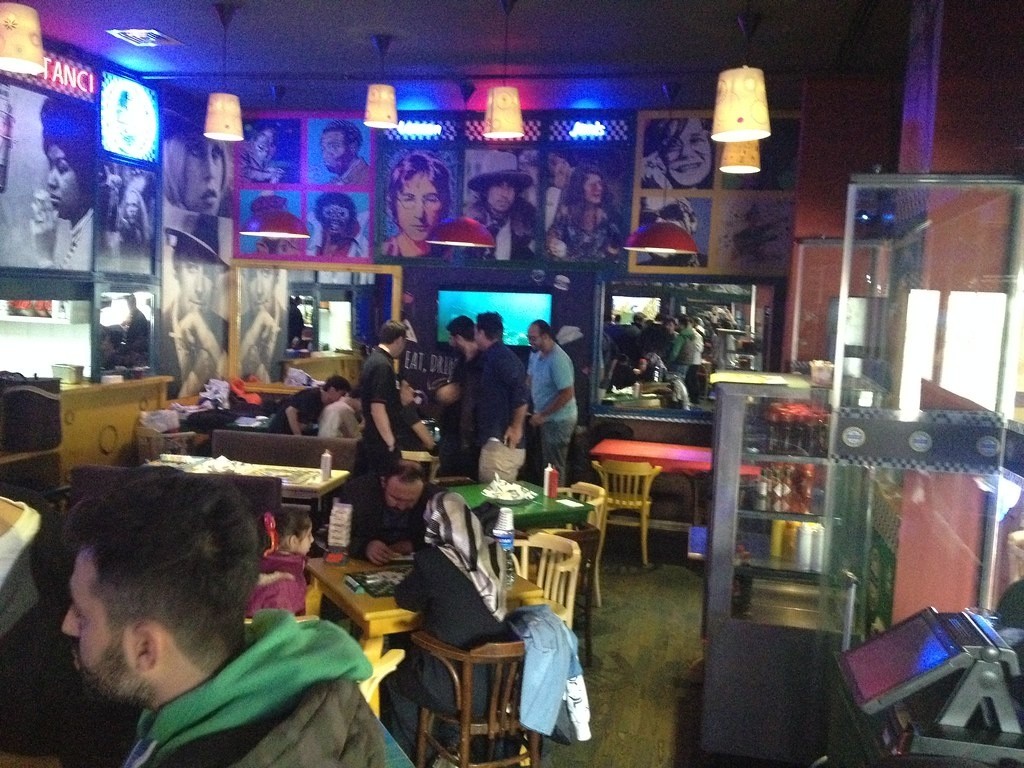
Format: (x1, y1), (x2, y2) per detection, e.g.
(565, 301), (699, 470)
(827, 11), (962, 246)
(228, 259), (403, 396)
(587, 271), (784, 425)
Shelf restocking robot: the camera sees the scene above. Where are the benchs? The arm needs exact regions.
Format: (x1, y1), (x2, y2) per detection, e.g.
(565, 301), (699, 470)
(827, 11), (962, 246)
(72, 466), (282, 546)
(211, 429), (359, 473)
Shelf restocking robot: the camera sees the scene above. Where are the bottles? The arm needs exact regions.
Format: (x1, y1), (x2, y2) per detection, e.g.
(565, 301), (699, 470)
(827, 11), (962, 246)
(543, 463), (553, 496)
(548, 467), (558, 497)
(492, 530), (513, 590)
(755, 496), (811, 515)
(748, 400), (829, 456)
(754, 463), (816, 498)
(320, 450), (331, 482)
(769, 520), (824, 573)
(633, 380), (644, 398)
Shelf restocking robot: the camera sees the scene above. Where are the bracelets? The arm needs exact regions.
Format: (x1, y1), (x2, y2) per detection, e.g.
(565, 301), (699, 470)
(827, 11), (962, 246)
(387, 442), (395, 448)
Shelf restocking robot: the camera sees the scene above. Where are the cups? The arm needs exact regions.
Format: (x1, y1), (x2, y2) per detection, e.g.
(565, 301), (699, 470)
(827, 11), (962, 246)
(494, 507), (514, 530)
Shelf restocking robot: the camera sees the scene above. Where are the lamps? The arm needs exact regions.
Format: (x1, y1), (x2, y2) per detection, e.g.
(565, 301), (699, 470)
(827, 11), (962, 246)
(623, 82), (699, 257)
(363, 34), (398, 129)
(202, 4), (244, 141)
(482, 0), (524, 139)
(424, 81), (496, 247)
(711, 0), (773, 173)
(238, 86), (311, 239)
(0, 0), (45, 74)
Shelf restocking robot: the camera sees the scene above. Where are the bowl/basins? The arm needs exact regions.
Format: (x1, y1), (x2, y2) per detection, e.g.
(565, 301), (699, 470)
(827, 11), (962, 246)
(810, 364), (832, 385)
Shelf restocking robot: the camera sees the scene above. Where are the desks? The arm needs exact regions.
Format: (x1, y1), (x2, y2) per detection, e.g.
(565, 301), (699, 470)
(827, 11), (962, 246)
(138, 453), (351, 533)
(590, 439), (760, 532)
(305, 540), (543, 721)
(445, 480), (595, 530)
(683, 525), (772, 686)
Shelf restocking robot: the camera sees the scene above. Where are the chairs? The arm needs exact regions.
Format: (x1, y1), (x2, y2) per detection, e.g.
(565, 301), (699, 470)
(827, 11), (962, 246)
(411, 459), (662, 768)
(134, 427), (196, 463)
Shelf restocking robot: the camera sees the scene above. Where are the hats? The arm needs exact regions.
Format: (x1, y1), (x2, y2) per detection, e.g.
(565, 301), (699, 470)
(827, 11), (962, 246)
(467, 151), (534, 194)
(165, 213), (228, 267)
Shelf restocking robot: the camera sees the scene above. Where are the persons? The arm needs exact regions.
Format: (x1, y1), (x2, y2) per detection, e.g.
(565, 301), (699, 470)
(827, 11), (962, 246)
(0, 310), (739, 767)
(29, 97), (291, 401)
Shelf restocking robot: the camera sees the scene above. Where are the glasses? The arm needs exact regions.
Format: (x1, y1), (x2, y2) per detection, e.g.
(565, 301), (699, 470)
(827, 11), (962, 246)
(395, 192), (440, 210)
(527, 334), (543, 341)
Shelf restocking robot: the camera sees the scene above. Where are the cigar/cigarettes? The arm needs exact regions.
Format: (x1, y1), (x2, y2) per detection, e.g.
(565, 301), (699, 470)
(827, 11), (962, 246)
(168, 331), (181, 338)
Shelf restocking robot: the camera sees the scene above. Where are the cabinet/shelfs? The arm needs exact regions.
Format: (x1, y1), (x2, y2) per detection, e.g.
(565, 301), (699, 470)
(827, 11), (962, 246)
(0, 298), (91, 325)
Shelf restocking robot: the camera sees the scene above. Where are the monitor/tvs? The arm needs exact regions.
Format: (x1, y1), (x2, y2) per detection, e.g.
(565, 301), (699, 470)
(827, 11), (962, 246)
(834, 605), (973, 715)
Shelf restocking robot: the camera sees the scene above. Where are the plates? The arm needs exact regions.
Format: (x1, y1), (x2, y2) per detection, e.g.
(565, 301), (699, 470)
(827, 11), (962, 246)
(481, 487), (530, 505)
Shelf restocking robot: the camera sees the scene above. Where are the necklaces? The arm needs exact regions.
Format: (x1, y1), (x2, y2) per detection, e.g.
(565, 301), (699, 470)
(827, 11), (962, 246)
(53, 230), (84, 268)
